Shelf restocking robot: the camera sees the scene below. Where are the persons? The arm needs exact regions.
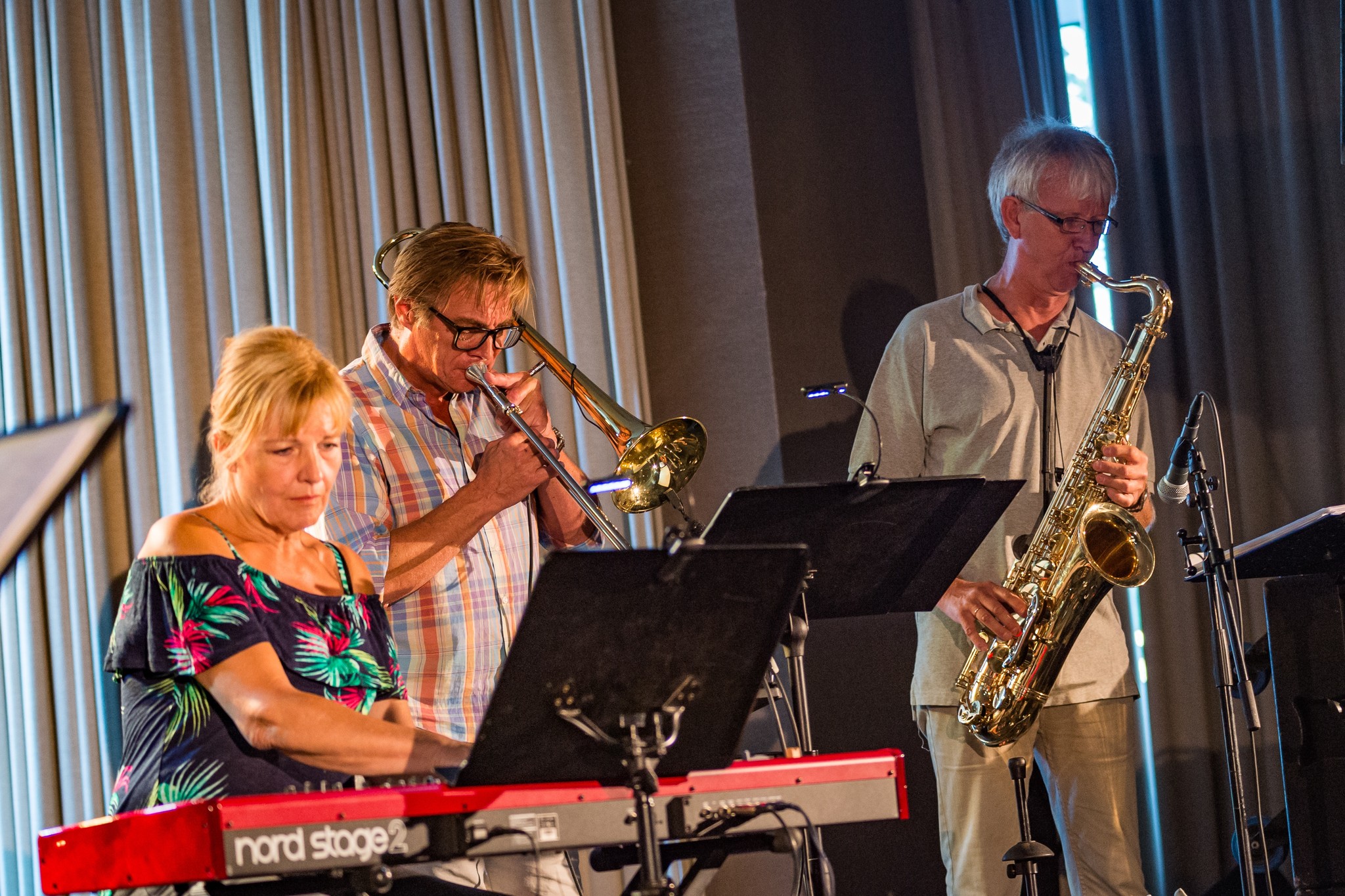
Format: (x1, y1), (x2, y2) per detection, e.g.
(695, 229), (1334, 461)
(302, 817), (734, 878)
(846, 114), (1156, 896)
(102, 324), (473, 896)
(305, 221), (605, 896)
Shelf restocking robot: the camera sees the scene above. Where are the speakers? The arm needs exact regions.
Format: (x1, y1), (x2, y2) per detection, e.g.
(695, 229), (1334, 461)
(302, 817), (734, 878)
(1263, 571), (1345, 896)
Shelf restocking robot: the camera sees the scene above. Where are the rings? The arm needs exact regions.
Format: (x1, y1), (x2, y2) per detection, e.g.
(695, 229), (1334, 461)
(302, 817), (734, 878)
(974, 607), (983, 616)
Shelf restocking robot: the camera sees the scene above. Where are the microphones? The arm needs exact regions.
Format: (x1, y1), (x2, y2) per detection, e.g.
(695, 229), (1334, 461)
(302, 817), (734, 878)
(1156, 395), (1205, 505)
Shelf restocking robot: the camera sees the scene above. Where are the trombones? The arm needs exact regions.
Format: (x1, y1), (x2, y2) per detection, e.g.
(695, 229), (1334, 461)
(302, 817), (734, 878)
(375, 228), (707, 548)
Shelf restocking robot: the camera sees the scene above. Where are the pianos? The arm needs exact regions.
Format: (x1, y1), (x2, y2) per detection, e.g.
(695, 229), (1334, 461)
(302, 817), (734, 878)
(39, 746), (911, 896)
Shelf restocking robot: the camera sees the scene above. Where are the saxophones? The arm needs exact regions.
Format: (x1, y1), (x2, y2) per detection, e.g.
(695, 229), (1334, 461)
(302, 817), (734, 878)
(957, 261), (1176, 748)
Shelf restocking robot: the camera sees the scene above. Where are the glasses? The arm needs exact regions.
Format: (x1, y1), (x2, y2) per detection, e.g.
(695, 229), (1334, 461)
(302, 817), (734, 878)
(1012, 194), (1118, 235)
(428, 306), (527, 351)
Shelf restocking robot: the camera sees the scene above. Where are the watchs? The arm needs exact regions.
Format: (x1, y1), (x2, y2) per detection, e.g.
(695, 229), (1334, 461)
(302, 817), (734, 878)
(550, 425), (565, 453)
(1125, 483), (1149, 514)
